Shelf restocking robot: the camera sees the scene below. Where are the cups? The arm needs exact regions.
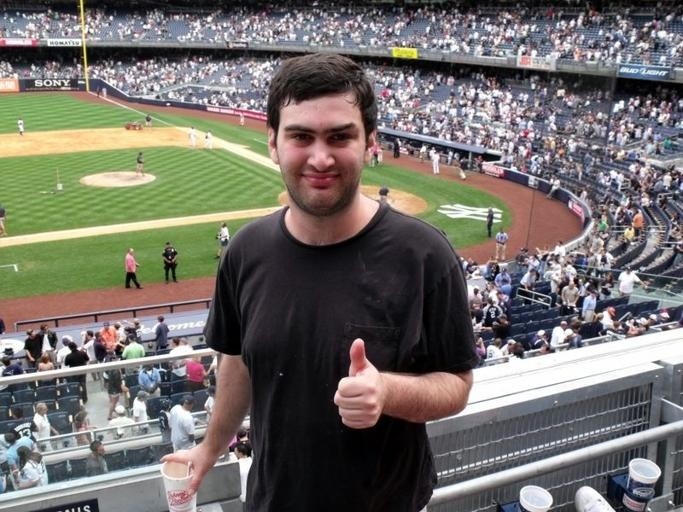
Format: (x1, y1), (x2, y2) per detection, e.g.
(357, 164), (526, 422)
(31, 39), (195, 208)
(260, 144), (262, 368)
(621, 458), (660, 511)
(518, 483), (554, 512)
(160, 460), (196, 512)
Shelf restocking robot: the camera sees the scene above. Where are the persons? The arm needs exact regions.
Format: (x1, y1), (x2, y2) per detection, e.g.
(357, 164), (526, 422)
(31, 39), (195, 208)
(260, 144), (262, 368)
(0, 0), (346, 154)
(1, 315), (253, 508)
(123, 245), (144, 291)
(160, 238), (178, 284)
(213, 224), (232, 261)
(136, 148), (147, 185)
(157, 50), (483, 511)
(0, 203), (7, 243)
(346, 2), (682, 366)
